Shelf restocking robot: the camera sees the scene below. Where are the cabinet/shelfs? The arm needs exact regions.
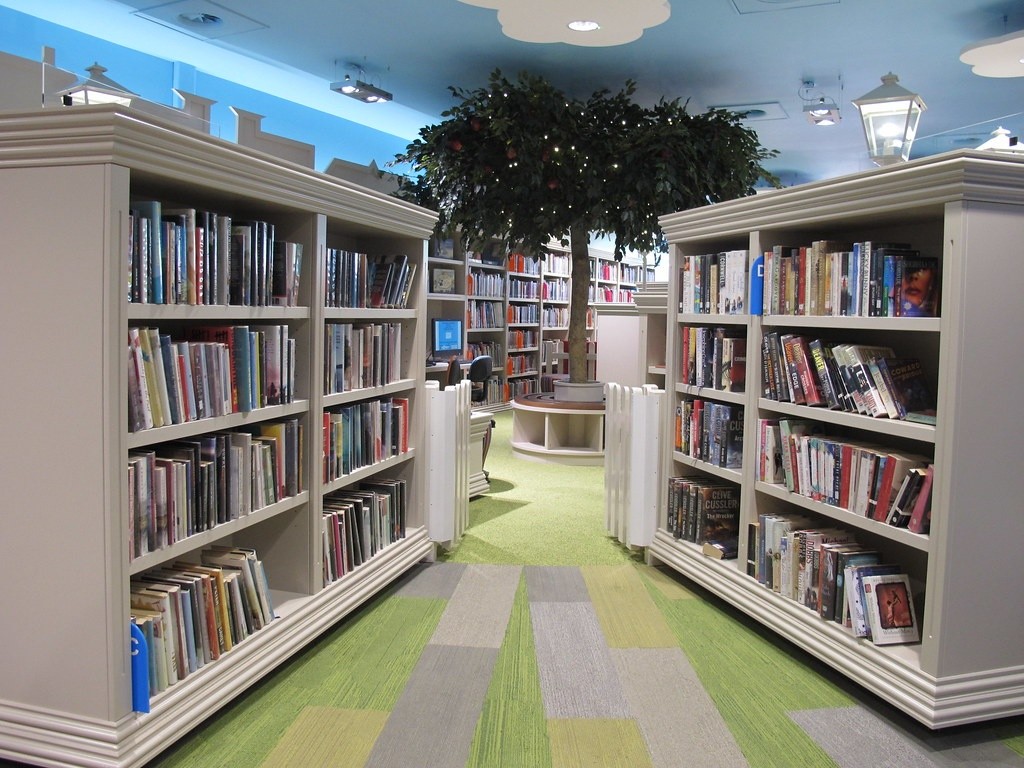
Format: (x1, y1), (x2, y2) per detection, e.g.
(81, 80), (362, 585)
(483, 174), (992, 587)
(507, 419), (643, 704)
(0, 104), (1024, 768)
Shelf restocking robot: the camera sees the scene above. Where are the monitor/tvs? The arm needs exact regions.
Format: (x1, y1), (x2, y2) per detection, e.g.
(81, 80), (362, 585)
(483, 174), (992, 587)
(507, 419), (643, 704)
(431, 318), (464, 357)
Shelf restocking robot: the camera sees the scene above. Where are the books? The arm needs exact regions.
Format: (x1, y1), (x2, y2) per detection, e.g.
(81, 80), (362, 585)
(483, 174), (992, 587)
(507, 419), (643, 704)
(124, 169), (572, 712)
(586, 241), (941, 647)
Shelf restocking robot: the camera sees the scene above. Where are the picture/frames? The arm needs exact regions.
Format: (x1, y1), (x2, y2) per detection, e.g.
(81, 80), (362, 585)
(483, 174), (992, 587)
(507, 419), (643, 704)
(432, 267), (457, 293)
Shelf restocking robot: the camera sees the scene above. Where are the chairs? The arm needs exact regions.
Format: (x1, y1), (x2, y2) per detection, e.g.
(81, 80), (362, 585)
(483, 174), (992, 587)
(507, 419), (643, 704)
(442, 355), (460, 391)
(467, 355), (492, 403)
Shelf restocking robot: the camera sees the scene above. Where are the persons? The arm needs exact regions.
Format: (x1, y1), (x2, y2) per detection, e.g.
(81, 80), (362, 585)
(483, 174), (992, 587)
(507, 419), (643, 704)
(883, 588), (903, 628)
(901, 268), (933, 308)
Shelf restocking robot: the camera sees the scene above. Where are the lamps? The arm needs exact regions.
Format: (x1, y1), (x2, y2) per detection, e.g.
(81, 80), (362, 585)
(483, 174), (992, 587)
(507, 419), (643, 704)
(802, 104), (840, 126)
(852, 74), (928, 166)
(975, 126), (1024, 155)
(54, 65), (133, 107)
(329, 65), (393, 104)
